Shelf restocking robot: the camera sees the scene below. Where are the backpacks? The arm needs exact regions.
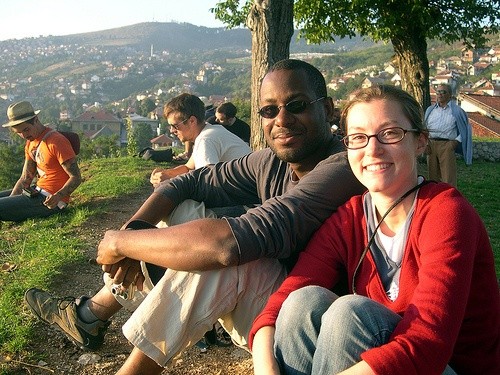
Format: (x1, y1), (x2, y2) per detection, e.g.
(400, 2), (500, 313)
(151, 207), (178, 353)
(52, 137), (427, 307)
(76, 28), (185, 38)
(138, 148), (172, 163)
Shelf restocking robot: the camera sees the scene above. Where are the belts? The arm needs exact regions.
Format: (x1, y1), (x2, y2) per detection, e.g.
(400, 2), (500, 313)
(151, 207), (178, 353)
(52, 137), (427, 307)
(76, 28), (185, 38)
(428, 137), (450, 141)
(35, 187), (66, 210)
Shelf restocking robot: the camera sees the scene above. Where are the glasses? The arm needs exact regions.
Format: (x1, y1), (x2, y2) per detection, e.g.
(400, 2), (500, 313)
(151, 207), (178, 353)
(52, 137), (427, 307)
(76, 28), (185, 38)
(436, 90), (450, 95)
(340, 126), (419, 149)
(257, 97), (326, 119)
(165, 118), (189, 130)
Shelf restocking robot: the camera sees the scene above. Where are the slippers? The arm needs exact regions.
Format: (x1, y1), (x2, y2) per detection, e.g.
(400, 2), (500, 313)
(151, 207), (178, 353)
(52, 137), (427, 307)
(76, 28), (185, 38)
(173, 152), (190, 160)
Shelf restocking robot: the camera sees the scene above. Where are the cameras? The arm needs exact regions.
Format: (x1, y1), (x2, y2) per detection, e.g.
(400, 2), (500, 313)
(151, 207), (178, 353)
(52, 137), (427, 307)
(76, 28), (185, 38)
(24, 187), (40, 198)
(111, 281), (137, 300)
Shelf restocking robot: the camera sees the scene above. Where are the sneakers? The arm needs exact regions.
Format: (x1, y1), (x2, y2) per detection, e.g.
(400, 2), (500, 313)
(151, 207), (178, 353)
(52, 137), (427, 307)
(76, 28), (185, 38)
(24, 288), (110, 353)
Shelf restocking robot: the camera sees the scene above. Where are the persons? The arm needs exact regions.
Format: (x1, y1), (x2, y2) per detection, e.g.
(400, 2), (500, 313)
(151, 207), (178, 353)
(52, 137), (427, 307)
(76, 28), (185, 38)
(24, 58), (362, 375)
(425, 83), (472, 188)
(205, 101), (251, 146)
(173, 96), (214, 160)
(149, 93), (253, 188)
(248, 84), (500, 375)
(0, 100), (83, 221)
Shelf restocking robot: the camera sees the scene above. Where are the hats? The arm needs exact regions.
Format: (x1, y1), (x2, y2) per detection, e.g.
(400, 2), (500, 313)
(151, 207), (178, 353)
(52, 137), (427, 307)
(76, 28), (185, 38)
(1, 101), (41, 128)
(103, 220), (170, 311)
(200, 96), (217, 112)
